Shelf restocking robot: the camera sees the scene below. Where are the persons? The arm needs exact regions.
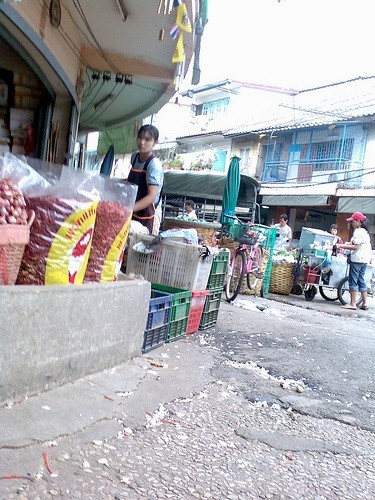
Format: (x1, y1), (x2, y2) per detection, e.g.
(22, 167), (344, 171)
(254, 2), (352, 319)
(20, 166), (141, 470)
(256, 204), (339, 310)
(127, 124), (164, 234)
(274, 213), (294, 238)
(336, 212), (373, 310)
(329, 223), (343, 253)
(182, 200), (197, 220)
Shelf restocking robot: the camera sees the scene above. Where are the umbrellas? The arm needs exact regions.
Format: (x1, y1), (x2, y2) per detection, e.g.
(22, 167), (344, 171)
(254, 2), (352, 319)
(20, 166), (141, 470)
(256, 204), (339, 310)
(220, 154), (241, 238)
(99, 143), (114, 178)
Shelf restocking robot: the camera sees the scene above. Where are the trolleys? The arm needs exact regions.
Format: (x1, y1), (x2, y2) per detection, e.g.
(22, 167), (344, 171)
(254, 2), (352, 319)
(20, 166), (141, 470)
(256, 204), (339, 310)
(319, 257), (375, 306)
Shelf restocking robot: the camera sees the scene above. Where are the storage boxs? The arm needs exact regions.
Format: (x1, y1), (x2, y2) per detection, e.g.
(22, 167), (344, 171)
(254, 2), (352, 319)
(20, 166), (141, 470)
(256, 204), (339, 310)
(126, 234), (229, 354)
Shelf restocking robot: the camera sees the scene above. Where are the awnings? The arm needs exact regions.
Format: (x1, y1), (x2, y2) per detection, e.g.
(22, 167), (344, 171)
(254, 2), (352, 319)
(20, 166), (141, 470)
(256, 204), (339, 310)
(336, 189), (375, 214)
(259, 182), (340, 207)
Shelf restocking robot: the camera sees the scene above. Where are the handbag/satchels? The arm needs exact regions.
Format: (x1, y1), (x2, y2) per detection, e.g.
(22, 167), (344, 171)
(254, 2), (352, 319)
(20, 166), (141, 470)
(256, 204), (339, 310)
(347, 254), (351, 263)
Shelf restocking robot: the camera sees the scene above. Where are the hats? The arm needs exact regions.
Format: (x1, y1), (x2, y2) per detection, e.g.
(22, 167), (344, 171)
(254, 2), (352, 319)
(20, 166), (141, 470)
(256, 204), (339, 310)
(346, 212), (366, 222)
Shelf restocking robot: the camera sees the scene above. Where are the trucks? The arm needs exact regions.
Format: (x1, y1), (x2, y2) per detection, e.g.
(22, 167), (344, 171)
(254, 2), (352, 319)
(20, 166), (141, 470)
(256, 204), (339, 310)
(162, 170), (260, 230)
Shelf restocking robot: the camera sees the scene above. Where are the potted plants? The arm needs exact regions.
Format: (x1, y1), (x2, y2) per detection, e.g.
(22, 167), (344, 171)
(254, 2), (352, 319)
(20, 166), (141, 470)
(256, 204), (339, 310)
(165, 159), (183, 170)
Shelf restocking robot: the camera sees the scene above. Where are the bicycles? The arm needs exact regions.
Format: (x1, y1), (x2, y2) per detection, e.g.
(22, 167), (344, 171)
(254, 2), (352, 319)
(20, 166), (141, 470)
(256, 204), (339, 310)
(222, 213), (271, 302)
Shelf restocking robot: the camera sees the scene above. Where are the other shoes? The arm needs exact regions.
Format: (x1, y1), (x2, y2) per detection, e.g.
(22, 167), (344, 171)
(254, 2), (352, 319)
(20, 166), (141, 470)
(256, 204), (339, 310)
(360, 305), (368, 310)
(341, 303), (357, 310)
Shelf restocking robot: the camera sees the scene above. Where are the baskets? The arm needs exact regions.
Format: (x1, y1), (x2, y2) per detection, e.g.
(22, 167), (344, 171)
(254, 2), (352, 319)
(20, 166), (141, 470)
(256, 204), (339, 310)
(166, 217), (222, 247)
(234, 224), (262, 245)
